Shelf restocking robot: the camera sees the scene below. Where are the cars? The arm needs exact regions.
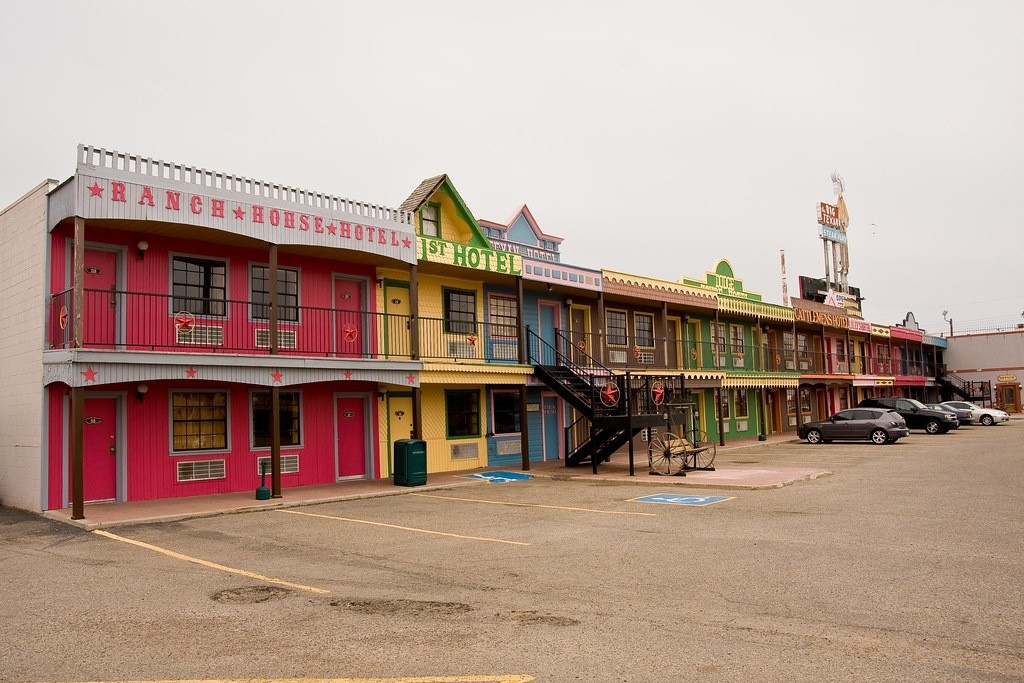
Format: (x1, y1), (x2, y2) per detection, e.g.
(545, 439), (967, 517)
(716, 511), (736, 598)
(798, 408), (910, 445)
(939, 401), (1010, 426)
(924, 403), (976, 425)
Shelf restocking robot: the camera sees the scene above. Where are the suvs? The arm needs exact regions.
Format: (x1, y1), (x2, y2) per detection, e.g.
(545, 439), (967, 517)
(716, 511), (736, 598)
(855, 395), (959, 434)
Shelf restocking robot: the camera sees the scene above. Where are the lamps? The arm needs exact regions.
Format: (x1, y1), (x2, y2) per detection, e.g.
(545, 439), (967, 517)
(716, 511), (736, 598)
(685, 314), (689, 324)
(378, 385), (387, 401)
(377, 274), (384, 287)
(765, 326), (769, 334)
(136, 384), (148, 399)
(137, 241), (149, 261)
(566, 298), (572, 313)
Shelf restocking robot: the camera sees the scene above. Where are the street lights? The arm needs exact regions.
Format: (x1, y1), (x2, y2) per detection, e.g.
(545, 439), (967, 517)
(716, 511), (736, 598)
(942, 309), (953, 336)
